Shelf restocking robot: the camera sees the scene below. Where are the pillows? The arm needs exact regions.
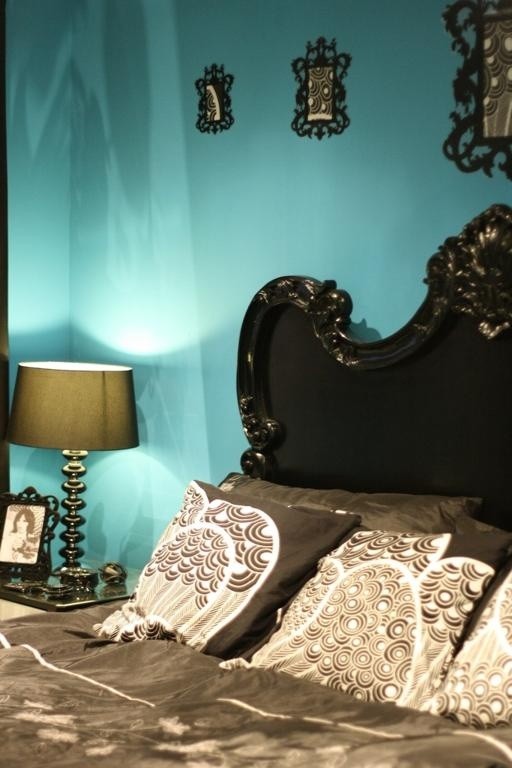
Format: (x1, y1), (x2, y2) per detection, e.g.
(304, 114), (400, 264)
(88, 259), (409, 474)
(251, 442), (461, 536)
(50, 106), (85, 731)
(421, 569), (512, 729)
(219, 523), (509, 720)
(97, 476), (364, 666)
(214, 472), (484, 543)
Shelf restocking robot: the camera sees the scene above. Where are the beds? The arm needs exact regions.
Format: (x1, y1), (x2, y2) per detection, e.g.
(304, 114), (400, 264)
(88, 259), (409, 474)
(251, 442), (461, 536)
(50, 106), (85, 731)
(0, 200), (510, 765)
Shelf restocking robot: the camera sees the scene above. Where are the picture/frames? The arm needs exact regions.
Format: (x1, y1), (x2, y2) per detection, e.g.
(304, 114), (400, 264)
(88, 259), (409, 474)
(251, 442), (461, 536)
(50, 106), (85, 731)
(1, 485), (60, 583)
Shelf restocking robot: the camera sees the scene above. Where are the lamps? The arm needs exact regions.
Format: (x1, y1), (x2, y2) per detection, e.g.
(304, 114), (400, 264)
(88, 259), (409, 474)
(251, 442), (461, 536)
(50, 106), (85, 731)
(6, 360), (141, 587)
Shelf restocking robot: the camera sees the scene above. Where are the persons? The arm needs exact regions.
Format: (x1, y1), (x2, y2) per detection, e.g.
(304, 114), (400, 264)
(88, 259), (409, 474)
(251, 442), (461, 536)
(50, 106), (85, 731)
(2, 509), (39, 563)
